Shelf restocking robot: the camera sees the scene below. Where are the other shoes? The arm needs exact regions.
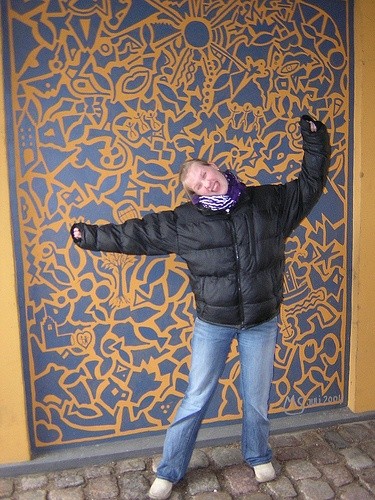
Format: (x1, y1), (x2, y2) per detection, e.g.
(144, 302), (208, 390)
(147, 478), (174, 500)
(252, 462), (277, 481)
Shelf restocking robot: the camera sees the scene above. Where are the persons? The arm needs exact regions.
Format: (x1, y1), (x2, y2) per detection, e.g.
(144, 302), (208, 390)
(70, 114), (331, 500)
